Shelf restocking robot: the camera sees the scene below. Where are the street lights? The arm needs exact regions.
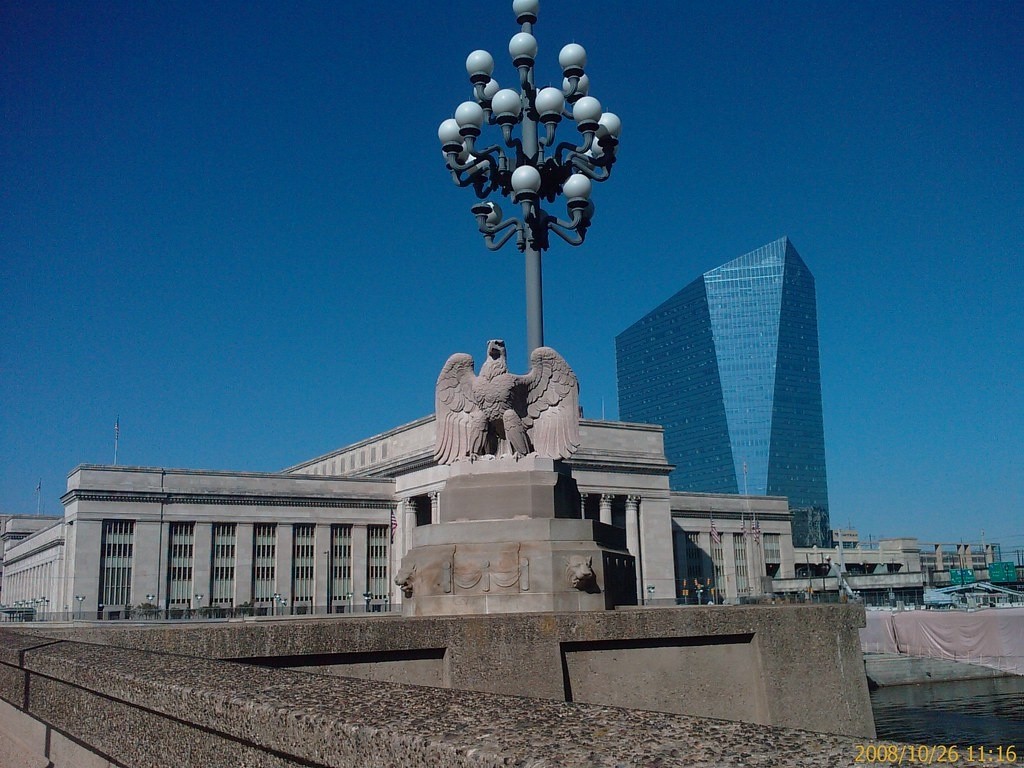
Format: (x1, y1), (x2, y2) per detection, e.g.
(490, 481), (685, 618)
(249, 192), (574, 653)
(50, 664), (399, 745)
(13, 596), (50, 621)
(75, 593), (86, 620)
(817, 552), (825, 593)
(1004, 563), (1009, 589)
(806, 551), (814, 600)
(363, 591), (371, 612)
(347, 592), (353, 614)
(274, 592), (288, 616)
(146, 593), (155, 620)
(438, 0), (621, 374)
(194, 593), (204, 620)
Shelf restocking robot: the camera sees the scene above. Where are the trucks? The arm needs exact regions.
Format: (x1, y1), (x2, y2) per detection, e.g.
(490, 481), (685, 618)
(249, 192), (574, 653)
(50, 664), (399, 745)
(923, 591), (957, 610)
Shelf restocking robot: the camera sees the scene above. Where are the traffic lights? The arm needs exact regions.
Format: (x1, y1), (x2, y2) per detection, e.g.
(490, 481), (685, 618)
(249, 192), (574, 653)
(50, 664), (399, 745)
(684, 579), (686, 586)
(682, 590), (689, 596)
(694, 579), (701, 590)
(708, 579), (711, 585)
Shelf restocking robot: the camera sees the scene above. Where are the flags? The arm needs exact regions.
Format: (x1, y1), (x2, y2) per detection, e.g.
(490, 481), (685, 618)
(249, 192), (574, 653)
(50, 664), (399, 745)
(34, 483), (40, 496)
(391, 511), (397, 543)
(115, 422), (120, 438)
(741, 516), (748, 539)
(752, 518), (761, 543)
(710, 518), (720, 544)
